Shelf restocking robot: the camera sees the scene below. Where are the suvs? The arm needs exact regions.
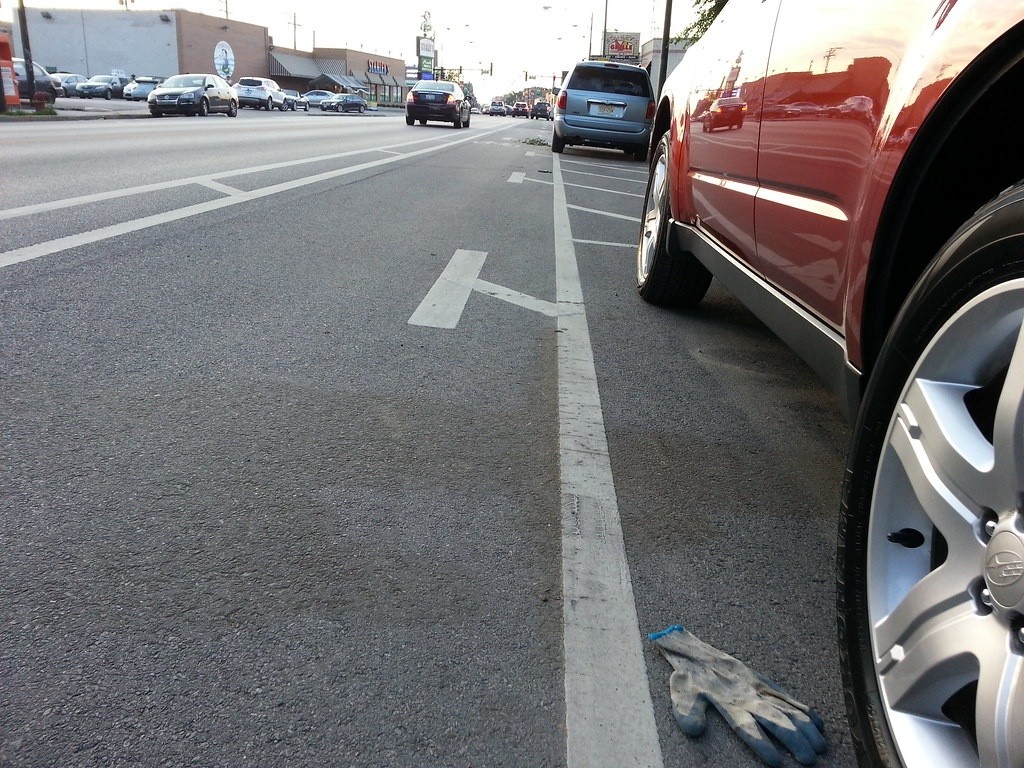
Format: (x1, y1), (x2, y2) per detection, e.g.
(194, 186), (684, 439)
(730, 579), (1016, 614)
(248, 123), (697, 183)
(702, 96), (748, 133)
(236, 76), (289, 113)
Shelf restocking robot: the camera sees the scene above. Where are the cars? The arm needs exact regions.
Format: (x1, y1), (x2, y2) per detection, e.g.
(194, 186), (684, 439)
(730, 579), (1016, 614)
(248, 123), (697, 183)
(639, 0), (1022, 767)
(550, 60), (657, 161)
(471, 100), (554, 121)
(405, 79), (472, 128)
(12, 58), (368, 117)
(754, 101), (826, 123)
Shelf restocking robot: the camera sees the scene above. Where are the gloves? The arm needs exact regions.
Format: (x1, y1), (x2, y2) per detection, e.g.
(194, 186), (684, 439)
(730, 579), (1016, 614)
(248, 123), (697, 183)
(649, 624), (828, 766)
